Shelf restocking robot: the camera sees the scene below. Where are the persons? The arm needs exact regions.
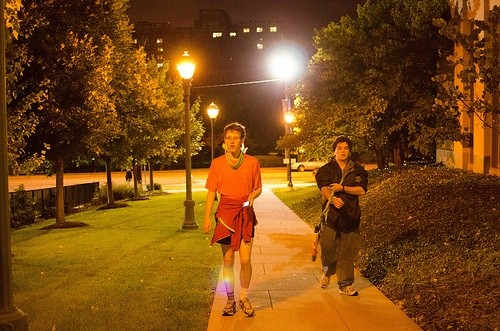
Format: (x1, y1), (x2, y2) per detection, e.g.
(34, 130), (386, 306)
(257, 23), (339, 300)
(126, 169), (133, 181)
(202, 121), (264, 317)
(315, 137), (368, 296)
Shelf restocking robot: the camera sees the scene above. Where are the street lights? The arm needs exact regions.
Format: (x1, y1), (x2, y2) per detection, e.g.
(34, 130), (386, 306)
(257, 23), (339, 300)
(283, 111), (295, 186)
(205, 102), (218, 201)
(175, 47), (200, 230)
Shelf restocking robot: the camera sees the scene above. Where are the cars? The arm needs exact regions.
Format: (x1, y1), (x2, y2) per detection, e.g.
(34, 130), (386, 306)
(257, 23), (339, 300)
(290, 157), (326, 171)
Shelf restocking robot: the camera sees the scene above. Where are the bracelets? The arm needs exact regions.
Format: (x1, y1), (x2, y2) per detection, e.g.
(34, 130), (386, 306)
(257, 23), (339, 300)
(342, 185), (345, 192)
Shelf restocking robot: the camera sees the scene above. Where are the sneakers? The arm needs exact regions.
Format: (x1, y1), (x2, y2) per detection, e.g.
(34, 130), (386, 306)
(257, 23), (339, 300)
(339, 285), (358, 296)
(223, 299), (236, 315)
(319, 275), (330, 288)
(239, 297), (255, 317)
